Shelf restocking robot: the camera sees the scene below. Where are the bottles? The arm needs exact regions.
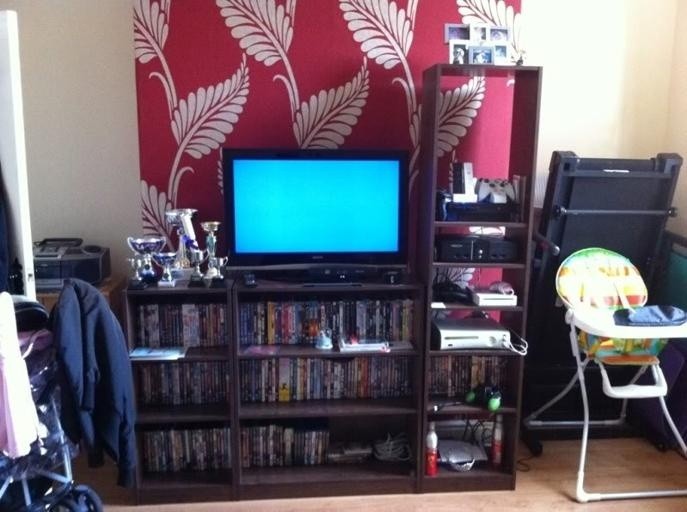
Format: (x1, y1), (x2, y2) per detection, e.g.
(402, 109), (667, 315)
(425, 422), (438, 476)
(491, 416), (504, 466)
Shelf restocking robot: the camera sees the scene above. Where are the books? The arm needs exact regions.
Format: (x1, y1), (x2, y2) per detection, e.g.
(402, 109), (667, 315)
(424, 292), (507, 400)
(240, 296), (417, 467)
(134, 302), (232, 475)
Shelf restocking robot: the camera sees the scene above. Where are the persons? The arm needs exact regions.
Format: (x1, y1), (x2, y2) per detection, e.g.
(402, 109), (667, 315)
(442, 21), (510, 67)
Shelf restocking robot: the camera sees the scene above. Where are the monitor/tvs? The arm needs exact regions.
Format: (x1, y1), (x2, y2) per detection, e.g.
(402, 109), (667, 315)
(220, 148), (412, 286)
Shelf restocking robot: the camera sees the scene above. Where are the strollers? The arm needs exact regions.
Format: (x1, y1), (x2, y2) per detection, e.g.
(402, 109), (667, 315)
(0, 278), (112, 511)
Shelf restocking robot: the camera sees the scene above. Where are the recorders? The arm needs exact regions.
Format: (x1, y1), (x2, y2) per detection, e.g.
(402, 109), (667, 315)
(32, 236), (111, 290)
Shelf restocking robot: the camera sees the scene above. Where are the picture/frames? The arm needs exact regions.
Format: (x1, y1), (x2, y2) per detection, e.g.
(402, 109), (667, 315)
(441, 22), (510, 67)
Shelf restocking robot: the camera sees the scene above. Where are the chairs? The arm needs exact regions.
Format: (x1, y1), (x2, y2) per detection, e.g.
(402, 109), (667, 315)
(522, 245), (687, 503)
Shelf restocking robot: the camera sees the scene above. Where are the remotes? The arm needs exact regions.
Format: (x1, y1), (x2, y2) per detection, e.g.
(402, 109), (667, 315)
(244, 271), (256, 286)
